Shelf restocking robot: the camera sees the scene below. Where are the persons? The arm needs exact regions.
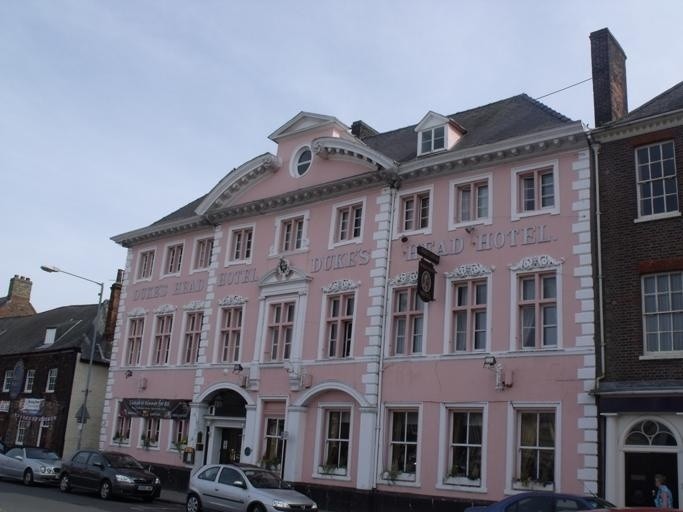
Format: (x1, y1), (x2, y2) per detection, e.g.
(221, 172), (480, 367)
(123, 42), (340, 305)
(652, 474), (673, 508)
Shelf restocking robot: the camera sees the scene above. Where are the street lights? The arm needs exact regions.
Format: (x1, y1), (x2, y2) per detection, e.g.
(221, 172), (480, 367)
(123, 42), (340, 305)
(38, 264), (106, 455)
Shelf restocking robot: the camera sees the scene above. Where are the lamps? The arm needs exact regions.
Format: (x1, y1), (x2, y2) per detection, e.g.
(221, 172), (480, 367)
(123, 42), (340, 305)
(232, 363), (248, 379)
(481, 356), (496, 370)
(123, 370), (140, 383)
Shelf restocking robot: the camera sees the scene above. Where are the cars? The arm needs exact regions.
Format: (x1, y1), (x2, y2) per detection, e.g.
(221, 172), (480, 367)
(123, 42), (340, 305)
(463, 480), (682, 512)
(0, 445), (62, 488)
(57, 449), (163, 502)
(184, 463), (317, 512)
